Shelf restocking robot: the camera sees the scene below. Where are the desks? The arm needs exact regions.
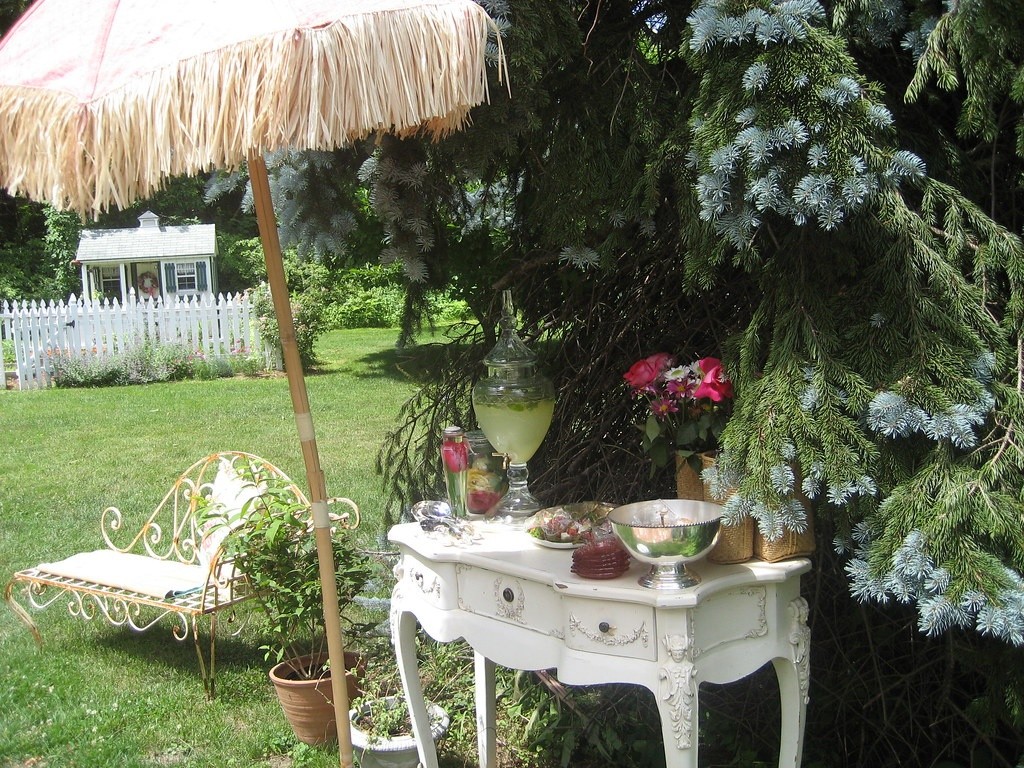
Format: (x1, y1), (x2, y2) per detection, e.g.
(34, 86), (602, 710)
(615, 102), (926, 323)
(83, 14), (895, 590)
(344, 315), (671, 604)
(387, 515), (814, 768)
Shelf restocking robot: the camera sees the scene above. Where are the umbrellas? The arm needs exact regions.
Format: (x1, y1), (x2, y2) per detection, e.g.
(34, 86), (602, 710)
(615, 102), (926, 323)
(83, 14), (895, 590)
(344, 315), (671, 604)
(0, 0), (490, 768)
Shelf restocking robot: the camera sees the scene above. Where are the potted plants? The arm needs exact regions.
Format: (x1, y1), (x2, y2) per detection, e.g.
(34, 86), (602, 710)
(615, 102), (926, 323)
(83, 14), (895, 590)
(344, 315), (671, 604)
(194, 485), (366, 749)
(316, 620), (476, 768)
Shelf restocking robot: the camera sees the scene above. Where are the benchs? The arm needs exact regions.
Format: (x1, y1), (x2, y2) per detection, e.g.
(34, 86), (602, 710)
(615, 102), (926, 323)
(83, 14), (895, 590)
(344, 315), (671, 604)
(4, 450), (359, 699)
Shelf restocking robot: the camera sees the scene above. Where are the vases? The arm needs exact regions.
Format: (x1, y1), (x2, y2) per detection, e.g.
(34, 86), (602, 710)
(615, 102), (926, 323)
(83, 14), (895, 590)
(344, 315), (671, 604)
(676, 450), (705, 501)
(754, 460), (817, 561)
(705, 451), (757, 564)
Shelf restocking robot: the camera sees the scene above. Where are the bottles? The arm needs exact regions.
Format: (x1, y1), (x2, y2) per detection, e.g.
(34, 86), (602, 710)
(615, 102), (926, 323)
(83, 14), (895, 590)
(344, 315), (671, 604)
(442, 425), (469, 519)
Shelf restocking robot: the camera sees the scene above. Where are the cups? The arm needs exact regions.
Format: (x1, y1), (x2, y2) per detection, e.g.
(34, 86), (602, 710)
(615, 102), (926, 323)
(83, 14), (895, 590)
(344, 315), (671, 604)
(465, 430), (509, 515)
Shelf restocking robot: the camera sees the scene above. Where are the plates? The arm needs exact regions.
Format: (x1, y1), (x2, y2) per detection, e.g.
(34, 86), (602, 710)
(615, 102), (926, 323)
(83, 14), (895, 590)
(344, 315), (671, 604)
(525, 502), (618, 549)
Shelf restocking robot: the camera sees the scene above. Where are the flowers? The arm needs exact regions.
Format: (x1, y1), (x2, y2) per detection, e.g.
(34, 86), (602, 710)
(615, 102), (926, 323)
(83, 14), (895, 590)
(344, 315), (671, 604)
(624, 354), (734, 478)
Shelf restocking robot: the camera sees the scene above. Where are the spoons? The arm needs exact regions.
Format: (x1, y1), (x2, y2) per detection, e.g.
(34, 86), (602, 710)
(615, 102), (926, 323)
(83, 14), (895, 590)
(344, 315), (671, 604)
(411, 500), (483, 546)
